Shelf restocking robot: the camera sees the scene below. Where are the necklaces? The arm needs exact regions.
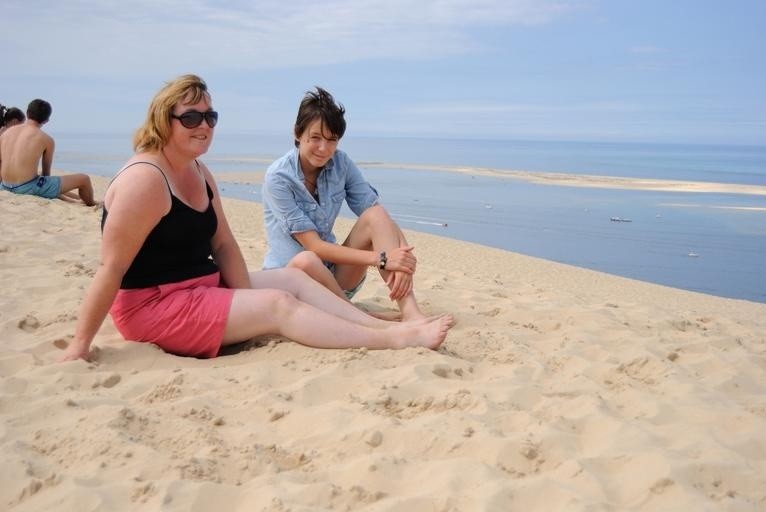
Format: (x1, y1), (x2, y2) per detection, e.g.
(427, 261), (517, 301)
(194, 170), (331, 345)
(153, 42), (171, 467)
(305, 179), (318, 196)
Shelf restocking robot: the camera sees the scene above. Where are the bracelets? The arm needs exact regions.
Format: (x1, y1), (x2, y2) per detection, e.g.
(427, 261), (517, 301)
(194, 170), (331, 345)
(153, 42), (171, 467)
(380, 250), (387, 269)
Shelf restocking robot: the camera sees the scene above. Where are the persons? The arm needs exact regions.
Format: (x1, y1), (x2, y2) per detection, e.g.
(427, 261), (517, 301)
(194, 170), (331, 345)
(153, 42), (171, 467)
(0, 99), (104, 206)
(0, 105), (82, 203)
(262, 86), (428, 322)
(61, 75), (455, 363)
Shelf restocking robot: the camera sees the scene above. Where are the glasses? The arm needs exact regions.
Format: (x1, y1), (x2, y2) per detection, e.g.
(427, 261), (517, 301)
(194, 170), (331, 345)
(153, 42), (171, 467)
(171, 112), (218, 129)
